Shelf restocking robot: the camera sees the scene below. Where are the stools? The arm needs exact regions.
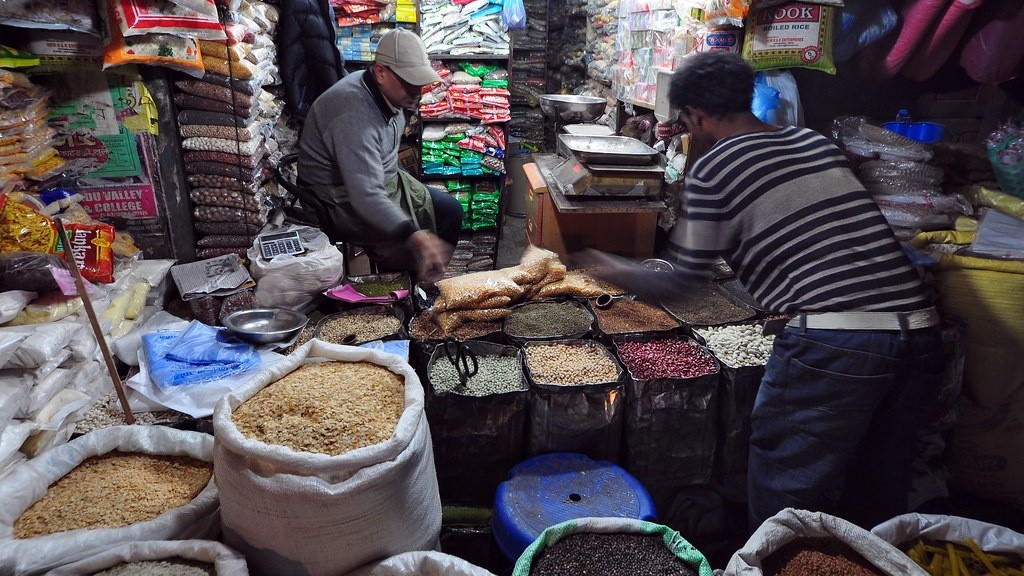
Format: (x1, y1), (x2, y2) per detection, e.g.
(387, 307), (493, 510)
(491, 453), (657, 565)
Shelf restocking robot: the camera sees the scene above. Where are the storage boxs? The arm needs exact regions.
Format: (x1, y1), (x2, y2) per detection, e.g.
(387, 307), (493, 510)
(398, 141), (423, 183)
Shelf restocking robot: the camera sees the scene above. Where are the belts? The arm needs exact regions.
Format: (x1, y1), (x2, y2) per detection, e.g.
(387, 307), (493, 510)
(786, 306), (941, 330)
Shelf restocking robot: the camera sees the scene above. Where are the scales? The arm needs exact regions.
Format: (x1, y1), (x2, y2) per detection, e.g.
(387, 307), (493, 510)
(551, 155), (664, 200)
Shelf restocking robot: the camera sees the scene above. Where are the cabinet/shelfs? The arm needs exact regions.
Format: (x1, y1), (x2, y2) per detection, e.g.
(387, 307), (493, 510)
(522, 163), (668, 265)
(416, 0), (549, 270)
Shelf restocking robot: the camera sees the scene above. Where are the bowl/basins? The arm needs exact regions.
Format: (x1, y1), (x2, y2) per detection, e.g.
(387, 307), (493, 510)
(539, 94), (607, 122)
(879, 120), (948, 145)
(223, 308), (308, 342)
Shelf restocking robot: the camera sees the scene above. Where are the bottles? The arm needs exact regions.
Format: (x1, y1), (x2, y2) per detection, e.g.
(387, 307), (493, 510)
(896, 110), (911, 124)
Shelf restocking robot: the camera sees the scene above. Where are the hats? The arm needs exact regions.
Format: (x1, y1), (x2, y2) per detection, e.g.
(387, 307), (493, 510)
(374, 28), (445, 86)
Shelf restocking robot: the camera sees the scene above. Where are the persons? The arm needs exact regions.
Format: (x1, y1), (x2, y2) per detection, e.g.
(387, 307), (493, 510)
(296, 27), (463, 280)
(589, 52), (941, 523)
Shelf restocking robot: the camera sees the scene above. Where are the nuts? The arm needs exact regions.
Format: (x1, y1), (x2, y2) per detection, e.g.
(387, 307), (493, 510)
(187, 175), (269, 224)
(698, 324), (776, 369)
(182, 137), (259, 156)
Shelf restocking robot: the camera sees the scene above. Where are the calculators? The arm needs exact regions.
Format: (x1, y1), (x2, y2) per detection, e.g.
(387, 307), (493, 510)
(259, 231), (305, 260)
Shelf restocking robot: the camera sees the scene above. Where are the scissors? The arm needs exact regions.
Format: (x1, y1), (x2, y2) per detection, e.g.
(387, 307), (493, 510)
(443, 337), (479, 388)
